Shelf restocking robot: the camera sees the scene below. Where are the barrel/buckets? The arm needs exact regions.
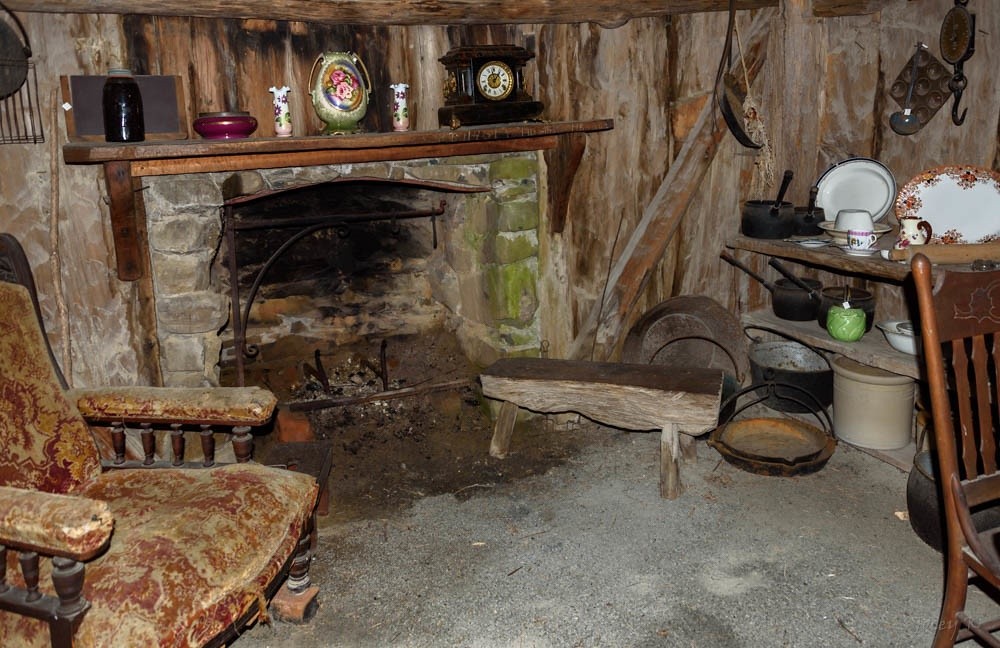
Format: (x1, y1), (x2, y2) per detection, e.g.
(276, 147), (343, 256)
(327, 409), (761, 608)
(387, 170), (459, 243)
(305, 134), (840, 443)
(744, 324), (833, 415)
(827, 353), (915, 449)
(647, 335), (746, 441)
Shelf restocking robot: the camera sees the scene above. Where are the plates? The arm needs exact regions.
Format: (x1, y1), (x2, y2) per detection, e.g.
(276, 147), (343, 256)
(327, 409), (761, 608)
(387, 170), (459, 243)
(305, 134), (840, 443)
(895, 165), (1000, 245)
(838, 245), (879, 256)
(814, 157), (898, 222)
(794, 238), (832, 249)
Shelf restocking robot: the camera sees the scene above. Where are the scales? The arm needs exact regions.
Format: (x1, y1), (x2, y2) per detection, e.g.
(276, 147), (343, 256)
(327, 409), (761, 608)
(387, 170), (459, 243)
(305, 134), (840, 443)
(939, 0), (976, 126)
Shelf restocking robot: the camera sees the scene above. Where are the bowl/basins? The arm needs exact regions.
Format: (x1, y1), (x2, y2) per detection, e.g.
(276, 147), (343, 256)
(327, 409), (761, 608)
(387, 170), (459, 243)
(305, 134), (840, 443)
(875, 319), (916, 355)
(834, 208), (874, 230)
(622, 292), (751, 386)
(817, 221), (893, 245)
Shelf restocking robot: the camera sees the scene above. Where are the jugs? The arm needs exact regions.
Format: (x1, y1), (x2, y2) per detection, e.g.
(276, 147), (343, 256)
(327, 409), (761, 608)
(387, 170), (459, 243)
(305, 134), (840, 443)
(893, 215), (932, 250)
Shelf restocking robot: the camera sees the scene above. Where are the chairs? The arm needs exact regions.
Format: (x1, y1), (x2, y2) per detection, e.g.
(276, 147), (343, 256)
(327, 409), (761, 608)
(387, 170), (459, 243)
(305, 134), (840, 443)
(0, 232), (320, 648)
(911, 252), (1000, 648)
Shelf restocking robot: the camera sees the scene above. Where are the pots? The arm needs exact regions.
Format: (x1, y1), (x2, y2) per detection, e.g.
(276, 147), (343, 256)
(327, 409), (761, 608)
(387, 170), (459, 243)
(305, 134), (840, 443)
(718, 5), (763, 148)
(768, 258), (874, 336)
(718, 249), (823, 321)
(793, 186), (825, 235)
(742, 170), (794, 240)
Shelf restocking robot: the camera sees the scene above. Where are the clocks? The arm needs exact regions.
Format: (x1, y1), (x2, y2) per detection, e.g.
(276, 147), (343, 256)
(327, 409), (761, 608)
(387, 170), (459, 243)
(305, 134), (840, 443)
(437, 44), (544, 131)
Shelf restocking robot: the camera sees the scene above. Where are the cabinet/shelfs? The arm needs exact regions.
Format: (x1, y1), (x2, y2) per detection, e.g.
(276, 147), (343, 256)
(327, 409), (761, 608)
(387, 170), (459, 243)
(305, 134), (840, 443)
(728, 232), (1000, 477)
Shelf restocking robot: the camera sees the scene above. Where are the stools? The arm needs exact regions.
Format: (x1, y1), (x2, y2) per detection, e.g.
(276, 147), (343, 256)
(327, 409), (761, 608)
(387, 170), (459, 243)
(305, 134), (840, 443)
(479, 357), (726, 501)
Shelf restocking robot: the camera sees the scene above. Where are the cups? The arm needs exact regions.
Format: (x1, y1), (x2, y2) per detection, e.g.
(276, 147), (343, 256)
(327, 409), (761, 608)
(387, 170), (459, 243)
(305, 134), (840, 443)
(846, 229), (877, 250)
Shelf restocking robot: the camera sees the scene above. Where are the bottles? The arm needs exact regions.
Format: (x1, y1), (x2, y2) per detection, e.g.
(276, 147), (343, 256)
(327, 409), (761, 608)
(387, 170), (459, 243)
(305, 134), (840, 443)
(269, 86), (292, 136)
(308, 51), (371, 134)
(390, 83), (410, 132)
(102, 69), (146, 142)
(192, 111), (258, 140)
